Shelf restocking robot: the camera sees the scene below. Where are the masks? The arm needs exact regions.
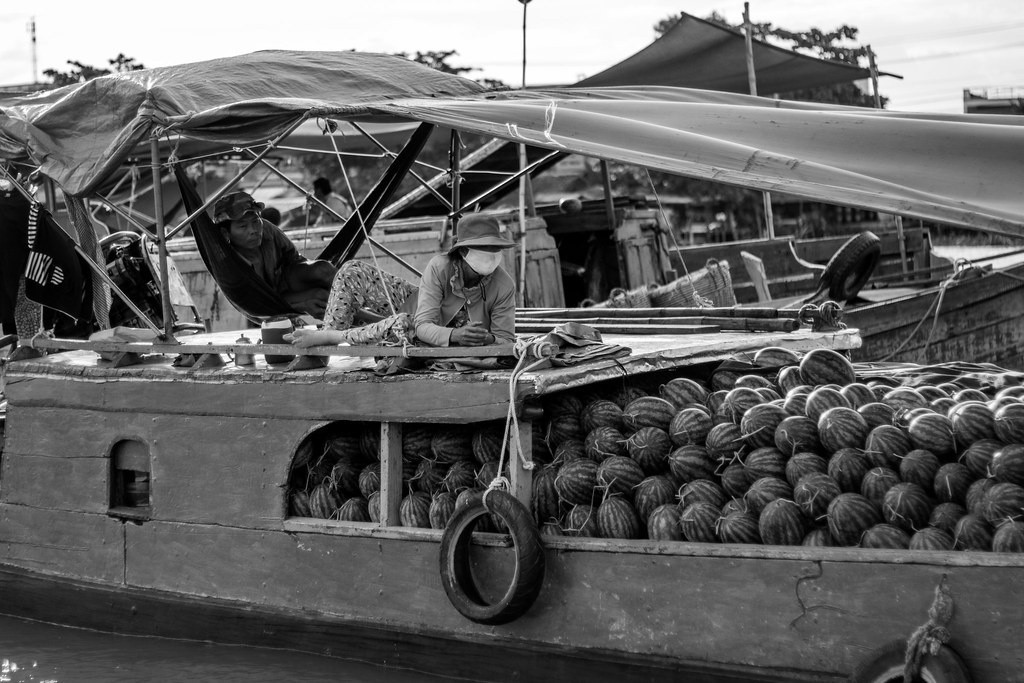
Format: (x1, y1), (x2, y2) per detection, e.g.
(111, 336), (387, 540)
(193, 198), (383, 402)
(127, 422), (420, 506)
(462, 246), (502, 276)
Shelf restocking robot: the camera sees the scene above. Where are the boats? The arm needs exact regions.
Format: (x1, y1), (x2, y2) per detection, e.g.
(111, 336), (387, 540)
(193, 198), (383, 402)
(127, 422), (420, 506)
(0, 47), (1024, 682)
(0, 0), (1024, 375)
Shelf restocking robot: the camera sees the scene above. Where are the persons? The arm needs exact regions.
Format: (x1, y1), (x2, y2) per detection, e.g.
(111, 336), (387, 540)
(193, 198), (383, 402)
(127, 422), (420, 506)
(308, 176), (354, 225)
(211, 191), (386, 327)
(282, 209), (517, 374)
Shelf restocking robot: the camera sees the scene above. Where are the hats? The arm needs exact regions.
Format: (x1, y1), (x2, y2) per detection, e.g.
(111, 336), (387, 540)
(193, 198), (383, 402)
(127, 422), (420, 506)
(448, 213), (516, 254)
(212, 191), (265, 224)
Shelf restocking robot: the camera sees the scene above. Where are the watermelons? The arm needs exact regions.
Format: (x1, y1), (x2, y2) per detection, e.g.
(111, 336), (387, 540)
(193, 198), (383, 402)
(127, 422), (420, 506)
(288, 344), (1023, 555)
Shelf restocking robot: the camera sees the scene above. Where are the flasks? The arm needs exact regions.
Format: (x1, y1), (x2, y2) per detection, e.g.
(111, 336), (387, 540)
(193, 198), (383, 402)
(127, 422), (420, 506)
(261, 316), (296, 364)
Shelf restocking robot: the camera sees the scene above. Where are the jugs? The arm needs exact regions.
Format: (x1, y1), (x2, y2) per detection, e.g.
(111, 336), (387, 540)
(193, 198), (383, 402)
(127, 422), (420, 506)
(227, 334), (263, 365)
